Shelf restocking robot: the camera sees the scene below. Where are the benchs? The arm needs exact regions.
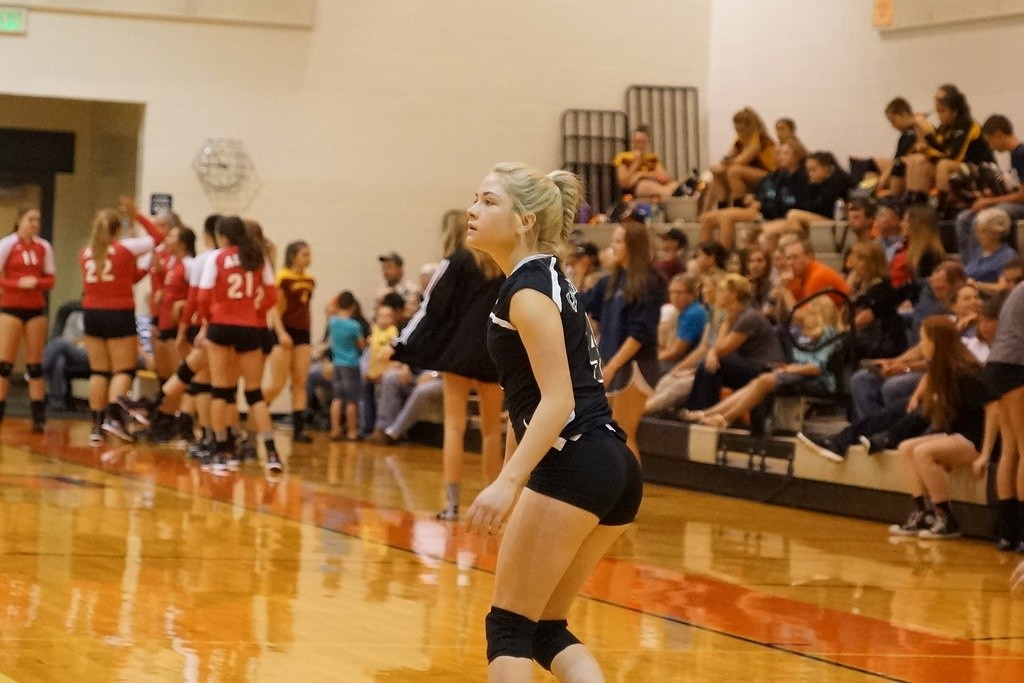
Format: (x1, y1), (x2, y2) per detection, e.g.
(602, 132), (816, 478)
(419, 185), (1024, 546)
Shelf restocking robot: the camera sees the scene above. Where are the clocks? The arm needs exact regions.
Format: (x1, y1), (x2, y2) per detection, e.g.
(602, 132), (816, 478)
(196, 137), (247, 190)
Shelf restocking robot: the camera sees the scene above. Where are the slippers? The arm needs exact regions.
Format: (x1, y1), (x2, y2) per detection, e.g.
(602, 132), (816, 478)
(329, 431), (363, 442)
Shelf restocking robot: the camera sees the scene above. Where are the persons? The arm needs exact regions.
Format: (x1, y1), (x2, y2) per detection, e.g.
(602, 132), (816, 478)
(313, 81), (1023, 553)
(467, 161), (642, 683)
(0, 206), (56, 435)
(24, 196), (316, 475)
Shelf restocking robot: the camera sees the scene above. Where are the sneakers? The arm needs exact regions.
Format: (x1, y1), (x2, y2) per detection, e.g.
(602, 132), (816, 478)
(797, 432), (847, 464)
(101, 413), (136, 442)
(184, 439), (256, 476)
(435, 503), (459, 521)
(859, 433), (889, 455)
(888, 508), (963, 539)
(267, 452), (283, 474)
(998, 537), (1024, 555)
(33, 420), (46, 434)
(292, 432), (312, 443)
(88, 426), (104, 442)
(116, 391), (151, 426)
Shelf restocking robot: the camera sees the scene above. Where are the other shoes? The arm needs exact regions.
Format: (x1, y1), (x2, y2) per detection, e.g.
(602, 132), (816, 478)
(677, 408), (732, 429)
(365, 425), (398, 444)
